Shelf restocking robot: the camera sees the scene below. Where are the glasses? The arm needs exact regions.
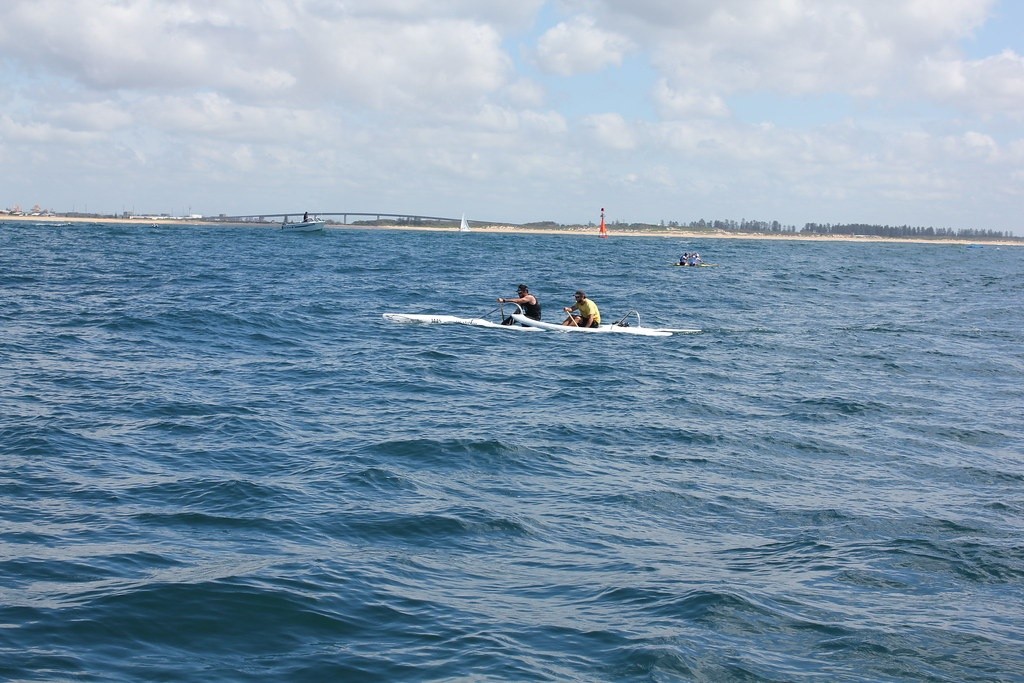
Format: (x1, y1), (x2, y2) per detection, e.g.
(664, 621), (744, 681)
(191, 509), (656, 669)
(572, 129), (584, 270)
(517, 291), (524, 294)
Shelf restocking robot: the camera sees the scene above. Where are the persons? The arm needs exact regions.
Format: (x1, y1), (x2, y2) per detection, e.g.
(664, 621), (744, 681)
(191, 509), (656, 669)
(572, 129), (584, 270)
(497, 284), (541, 328)
(561, 289), (601, 328)
(680, 253), (704, 266)
(303, 211), (309, 223)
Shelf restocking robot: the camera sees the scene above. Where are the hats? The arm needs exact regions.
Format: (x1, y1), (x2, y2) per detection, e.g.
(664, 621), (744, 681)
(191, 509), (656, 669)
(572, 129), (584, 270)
(572, 290), (586, 297)
(515, 284), (527, 291)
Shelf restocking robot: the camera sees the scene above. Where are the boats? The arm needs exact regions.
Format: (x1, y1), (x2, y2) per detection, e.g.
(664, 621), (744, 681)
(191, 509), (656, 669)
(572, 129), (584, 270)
(275, 220), (326, 232)
(510, 313), (703, 338)
(379, 301), (547, 333)
(677, 260), (719, 268)
